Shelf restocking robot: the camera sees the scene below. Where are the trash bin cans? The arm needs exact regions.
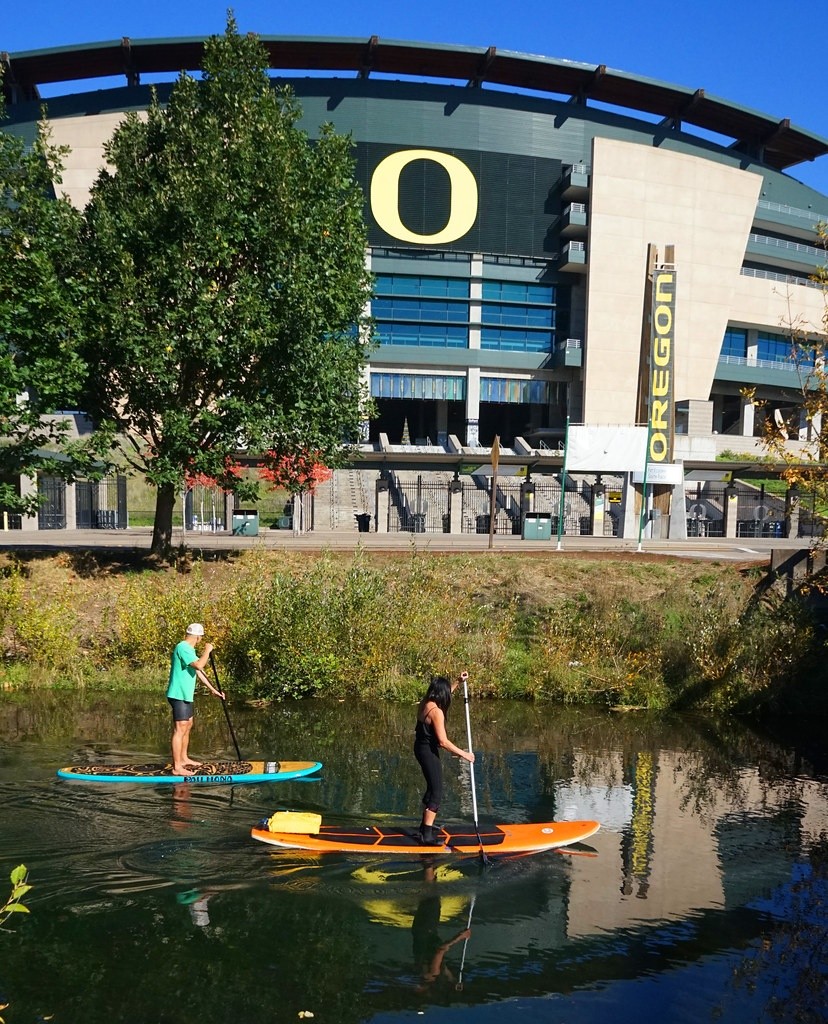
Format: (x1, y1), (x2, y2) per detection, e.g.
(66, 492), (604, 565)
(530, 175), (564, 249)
(521, 511), (552, 540)
(232, 509), (259, 537)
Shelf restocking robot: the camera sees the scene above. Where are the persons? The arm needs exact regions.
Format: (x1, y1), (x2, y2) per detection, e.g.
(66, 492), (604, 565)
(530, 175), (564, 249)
(165, 623), (226, 776)
(413, 670), (475, 843)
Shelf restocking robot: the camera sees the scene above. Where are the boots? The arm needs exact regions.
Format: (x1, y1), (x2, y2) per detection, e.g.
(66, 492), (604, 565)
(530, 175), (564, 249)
(421, 824), (443, 846)
(419, 818), (441, 835)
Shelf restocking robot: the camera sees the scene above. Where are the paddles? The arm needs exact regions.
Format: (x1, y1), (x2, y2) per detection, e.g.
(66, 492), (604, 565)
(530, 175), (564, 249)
(463, 680), (478, 826)
(455, 898), (475, 991)
(209, 651), (242, 762)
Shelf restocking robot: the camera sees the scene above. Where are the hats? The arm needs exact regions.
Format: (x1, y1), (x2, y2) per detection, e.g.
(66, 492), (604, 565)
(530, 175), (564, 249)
(185, 623), (205, 636)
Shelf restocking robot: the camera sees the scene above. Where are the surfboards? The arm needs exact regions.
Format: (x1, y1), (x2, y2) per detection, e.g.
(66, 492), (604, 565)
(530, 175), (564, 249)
(251, 816), (601, 853)
(56, 760), (322, 782)
